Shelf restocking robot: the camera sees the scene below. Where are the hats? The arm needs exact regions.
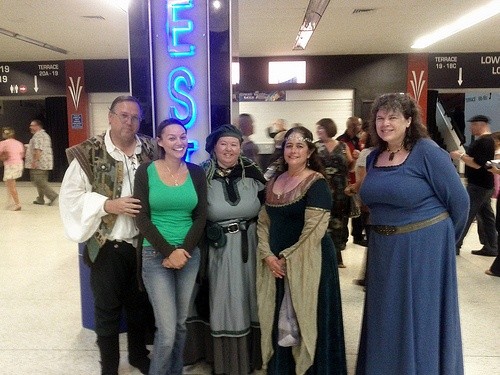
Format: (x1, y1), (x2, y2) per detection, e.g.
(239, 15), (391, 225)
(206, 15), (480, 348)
(465, 115), (489, 122)
(205, 123), (244, 153)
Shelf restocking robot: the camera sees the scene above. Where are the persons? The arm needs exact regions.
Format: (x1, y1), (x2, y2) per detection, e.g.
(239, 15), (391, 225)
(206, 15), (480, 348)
(0, 125), (28, 209)
(195, 123), (271, 375)
(58, 95), (160, 375)
(235, 106), (385, 286)
(353, 93), (475, 375)
(444, 115), (499, 255)
(133, 117), (210, 374)
(24, 120), (60, 204)
(253, 126), (346, 375)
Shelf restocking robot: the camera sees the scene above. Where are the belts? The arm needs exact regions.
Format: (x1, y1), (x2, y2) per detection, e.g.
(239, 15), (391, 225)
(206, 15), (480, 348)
(223, 215), (259, 234)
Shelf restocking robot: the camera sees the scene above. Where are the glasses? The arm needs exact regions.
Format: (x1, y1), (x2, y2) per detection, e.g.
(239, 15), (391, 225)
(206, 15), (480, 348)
(111, 110), (142, 124)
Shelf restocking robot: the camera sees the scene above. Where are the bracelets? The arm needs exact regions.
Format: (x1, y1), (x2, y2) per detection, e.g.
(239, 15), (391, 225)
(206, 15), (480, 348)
(458, 153), (466, 160)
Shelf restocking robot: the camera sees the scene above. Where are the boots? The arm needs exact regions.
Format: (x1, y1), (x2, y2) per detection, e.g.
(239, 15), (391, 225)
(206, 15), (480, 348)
(126, 316), (150, 372)
(94, 334), (119, 375)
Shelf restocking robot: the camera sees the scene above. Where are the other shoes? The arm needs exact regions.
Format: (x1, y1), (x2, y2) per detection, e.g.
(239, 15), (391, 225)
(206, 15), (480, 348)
(11, 205), (21, 211)
(353, 240), (369, 247)
(34, 201), (40, 204)
(472, 248), (496, 257)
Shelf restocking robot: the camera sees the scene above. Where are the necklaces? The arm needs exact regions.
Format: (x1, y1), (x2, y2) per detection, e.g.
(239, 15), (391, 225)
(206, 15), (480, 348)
(161, 160), (183, 186)
(386, 143), (405, 161)
(276, 170), (303, 199)
